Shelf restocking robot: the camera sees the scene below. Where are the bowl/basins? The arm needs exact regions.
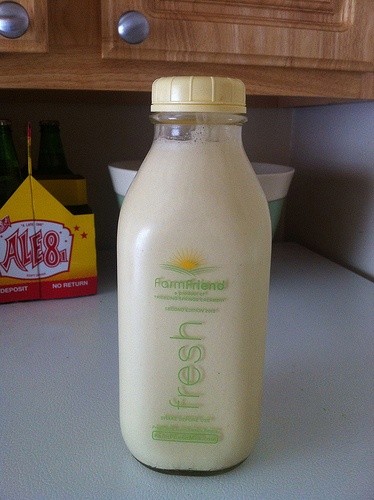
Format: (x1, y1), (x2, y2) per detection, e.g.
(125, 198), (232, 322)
(107, 159), (295, 235)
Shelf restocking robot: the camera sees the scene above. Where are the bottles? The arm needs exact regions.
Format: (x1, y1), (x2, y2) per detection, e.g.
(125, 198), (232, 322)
(35, 119), (93, 217)
(115, 76), (271, 473)
(0, 119), (23, 207)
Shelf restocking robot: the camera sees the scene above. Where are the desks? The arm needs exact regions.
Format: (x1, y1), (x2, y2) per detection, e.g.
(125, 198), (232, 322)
(0, 237), (374, 500)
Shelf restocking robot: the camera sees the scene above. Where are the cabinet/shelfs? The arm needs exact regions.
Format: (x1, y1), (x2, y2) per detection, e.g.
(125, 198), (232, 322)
(0, 0), (374, 106)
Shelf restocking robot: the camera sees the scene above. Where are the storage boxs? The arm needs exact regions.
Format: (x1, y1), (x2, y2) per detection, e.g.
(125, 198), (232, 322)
(0, 120), (99, 307)
(35, 176), (89, 206)
